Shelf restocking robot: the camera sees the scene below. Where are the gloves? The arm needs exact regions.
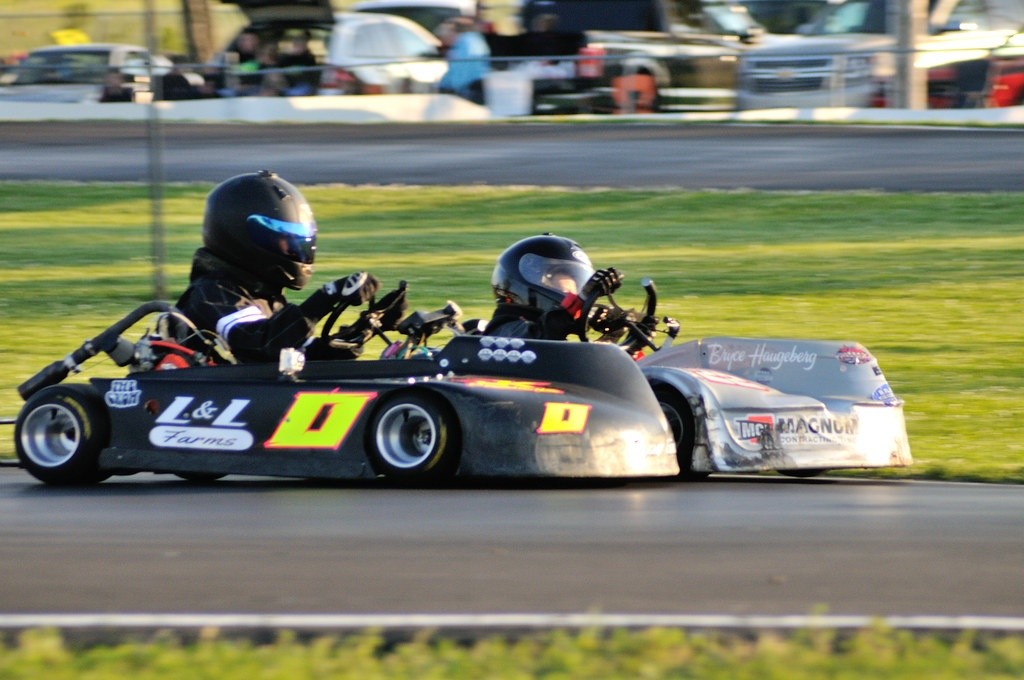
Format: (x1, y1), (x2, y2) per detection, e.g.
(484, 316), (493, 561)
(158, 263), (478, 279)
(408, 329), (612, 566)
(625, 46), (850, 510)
(324, 271), (381, 306)
(588, 305), (623, 337)
(582, 266), (621, 302)
(362, 289), (407, 331)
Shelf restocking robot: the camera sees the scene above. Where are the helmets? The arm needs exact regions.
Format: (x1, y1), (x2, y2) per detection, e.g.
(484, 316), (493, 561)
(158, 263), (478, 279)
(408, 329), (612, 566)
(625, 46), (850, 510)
(491, 233), (594, 334)
(202, 170), (316, 291)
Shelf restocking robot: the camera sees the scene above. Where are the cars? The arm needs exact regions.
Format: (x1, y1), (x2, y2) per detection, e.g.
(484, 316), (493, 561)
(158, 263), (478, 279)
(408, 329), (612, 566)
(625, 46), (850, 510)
(0, 43), (218, 106)
(223, 16), (448, 103)
(486, 0), (1022, 111)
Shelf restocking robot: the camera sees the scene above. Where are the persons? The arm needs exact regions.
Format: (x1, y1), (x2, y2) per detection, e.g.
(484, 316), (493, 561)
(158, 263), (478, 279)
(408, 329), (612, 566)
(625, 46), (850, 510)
(482, 232), (657, 355)
(89, 11), (660, 118)
(158, 171), (408, 362)
